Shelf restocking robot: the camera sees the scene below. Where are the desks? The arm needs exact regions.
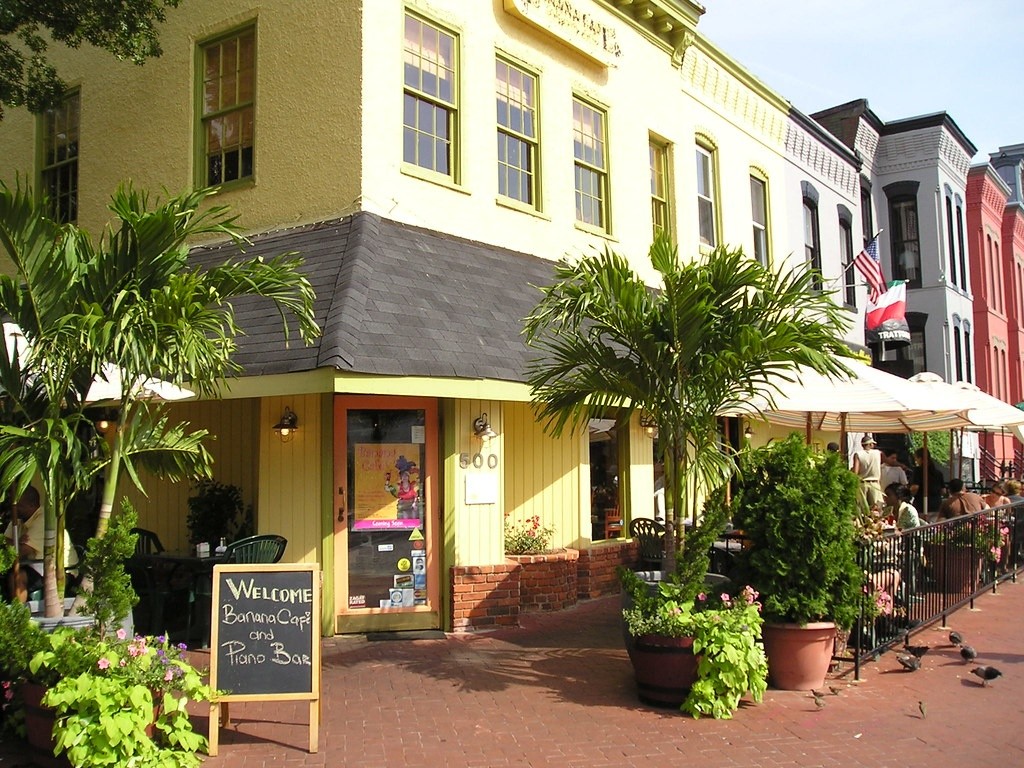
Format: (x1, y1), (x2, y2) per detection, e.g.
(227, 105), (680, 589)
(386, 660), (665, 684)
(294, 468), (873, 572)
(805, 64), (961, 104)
(708, 541), (746, 553)
(115, 552), (237, 646)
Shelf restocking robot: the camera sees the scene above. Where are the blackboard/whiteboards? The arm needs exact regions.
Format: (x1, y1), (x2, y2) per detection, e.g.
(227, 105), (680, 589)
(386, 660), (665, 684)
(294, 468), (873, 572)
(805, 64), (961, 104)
(209, 563), (320, 702)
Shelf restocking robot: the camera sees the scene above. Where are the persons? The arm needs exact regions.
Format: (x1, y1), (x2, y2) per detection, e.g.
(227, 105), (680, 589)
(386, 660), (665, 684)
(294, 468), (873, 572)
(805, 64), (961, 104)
(1003, 480), (1024, 514)
(852, 436), (906, 528)
(880, 449), (908, 496)
(883, 482), (920, 551)
(934, 478), (992, 523)
(4, 487), (79, 604)
(909, 447), (947, 514)
(827, 442), (839, 453)
(986, 482), (1011, 515)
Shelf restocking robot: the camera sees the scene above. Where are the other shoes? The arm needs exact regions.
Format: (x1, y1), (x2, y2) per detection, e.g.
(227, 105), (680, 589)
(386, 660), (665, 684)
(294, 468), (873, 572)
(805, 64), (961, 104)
(886, 614), (923, 630)
(876, 622), (909, 637)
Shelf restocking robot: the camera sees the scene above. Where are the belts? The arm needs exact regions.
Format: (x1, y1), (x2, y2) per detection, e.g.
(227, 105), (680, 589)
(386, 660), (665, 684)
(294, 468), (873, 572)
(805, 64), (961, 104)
(861, 480), (879, 483)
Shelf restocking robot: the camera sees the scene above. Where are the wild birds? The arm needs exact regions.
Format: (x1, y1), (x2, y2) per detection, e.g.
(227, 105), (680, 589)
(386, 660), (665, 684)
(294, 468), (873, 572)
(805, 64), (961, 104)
(809, 689), (827, 708)
(894, 644), (931, 671)
(970, 665), (1004, 686)
(918, 699), (928, 719)
(949, 630), (962, 647)
(959, 644), (977, 662)
(829, 686), (844, 695)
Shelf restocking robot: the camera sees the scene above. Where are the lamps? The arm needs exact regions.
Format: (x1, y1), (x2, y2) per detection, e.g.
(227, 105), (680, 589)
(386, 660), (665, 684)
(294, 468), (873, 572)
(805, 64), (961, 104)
(271, 405), (299, 443)
(642, 422), (658, 439)
(743, 420), (756, 438)
(474, 412), (497, 442)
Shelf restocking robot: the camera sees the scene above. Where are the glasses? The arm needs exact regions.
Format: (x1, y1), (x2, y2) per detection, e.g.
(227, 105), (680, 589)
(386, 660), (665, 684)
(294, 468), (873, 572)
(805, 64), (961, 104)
(991, 490), (1002, 496)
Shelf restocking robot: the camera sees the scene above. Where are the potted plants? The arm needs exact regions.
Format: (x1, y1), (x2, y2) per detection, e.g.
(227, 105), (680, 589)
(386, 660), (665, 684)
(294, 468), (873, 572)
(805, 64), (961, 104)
(0, 166), (323, 642)
(516, 227), (874, 719)
(26, 496), (234, 768)
(737, 432), (880, 691)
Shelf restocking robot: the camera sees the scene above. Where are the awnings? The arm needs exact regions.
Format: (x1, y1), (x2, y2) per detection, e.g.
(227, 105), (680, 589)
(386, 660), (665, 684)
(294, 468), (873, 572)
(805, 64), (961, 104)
(865, 316), (911, 351)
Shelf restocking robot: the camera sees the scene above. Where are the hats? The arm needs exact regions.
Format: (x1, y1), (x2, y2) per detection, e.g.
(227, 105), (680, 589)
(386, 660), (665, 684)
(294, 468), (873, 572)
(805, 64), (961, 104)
(861, 438), (877, 445)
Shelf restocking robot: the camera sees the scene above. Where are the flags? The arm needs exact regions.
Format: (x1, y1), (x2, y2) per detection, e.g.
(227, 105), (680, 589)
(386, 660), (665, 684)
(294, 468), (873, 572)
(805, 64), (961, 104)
(865, 279), (905, 329)
(854, 235), (887, 306)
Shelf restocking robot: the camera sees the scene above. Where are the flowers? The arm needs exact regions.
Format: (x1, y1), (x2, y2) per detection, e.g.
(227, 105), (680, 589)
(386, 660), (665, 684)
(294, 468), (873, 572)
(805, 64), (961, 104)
(934, 515), (1009, 564)
(655, 584), (770, 718)
(95, 627), (188, 694)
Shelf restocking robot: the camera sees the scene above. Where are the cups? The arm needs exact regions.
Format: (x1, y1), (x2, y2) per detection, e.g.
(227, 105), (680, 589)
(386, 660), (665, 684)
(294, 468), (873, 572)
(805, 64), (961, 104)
(889, 514), (896, 524)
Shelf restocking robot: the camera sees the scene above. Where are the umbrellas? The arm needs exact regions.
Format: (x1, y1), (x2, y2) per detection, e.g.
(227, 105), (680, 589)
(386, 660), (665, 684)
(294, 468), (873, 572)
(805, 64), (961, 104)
(677, 350), (1024, 495)
(0, 321), (196, 404)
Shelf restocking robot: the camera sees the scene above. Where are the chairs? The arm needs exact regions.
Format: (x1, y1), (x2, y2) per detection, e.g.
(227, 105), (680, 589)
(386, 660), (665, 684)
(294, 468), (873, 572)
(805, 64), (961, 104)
(706, 546), (736, 580)
(603, 508), (623, 540)
(629, 518), (668, 571)
(128, 528), (165, 555)
(187, 534), (288, 648)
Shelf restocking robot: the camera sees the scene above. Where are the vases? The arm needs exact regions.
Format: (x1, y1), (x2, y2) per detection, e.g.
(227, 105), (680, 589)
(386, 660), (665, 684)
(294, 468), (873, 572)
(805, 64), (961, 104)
(929, 544), (985, 594)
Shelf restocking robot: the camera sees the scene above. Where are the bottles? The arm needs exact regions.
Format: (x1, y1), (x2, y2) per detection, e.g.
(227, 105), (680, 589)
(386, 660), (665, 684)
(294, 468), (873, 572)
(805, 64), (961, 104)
(220, 536), (226, 547)
